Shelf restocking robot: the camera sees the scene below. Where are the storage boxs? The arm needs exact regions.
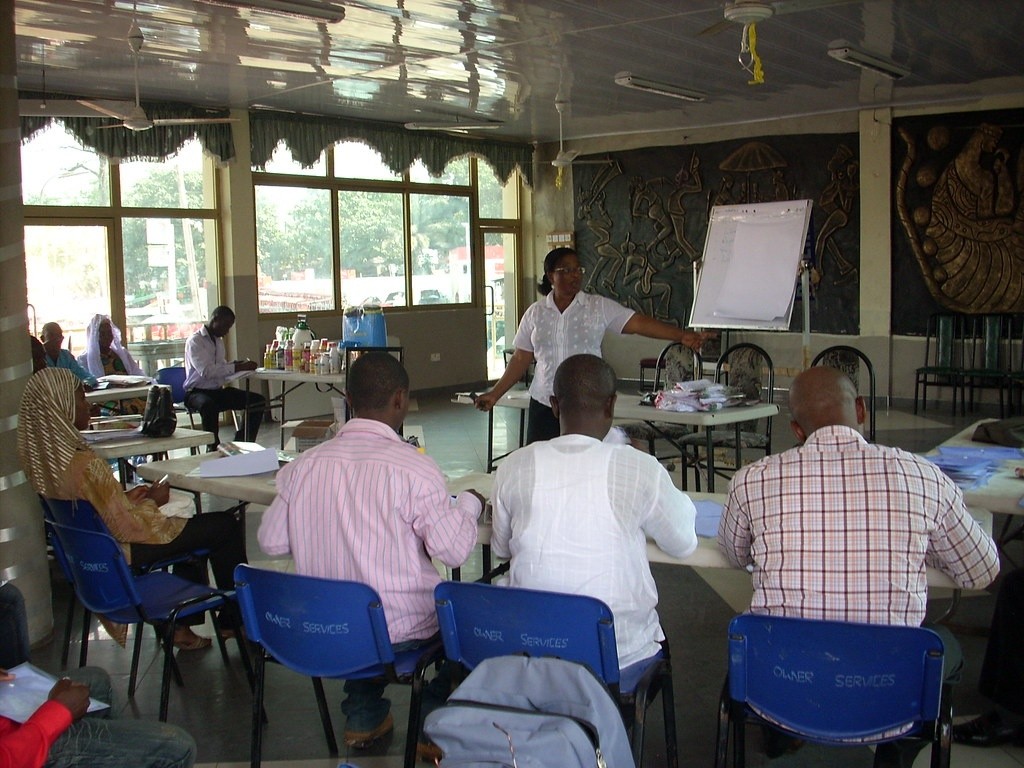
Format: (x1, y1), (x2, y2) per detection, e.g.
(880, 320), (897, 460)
(279, 419), (340, 455)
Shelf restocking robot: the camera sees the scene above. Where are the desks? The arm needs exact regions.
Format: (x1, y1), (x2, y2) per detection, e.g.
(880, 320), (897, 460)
(50, 364), (1024, 627)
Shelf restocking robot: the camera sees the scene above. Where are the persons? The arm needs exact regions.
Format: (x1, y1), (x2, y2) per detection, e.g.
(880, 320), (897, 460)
(949, 567), (1024, 748)
(77, 314), (157, 466)
(0, 665), (197, 768)
(183, 305), (265, 453)
(31, 321), (100, 417)
(474, 248), (717, 447)
(18, 367), (249, 649)
(718, 365), (999, 768)
(257, 352), (486, 762)
(489, 353), (696, 693)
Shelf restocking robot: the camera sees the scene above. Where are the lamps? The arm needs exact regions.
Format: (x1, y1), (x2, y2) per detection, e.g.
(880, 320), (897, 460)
(826, 38), (913, 81)
(613, 72), (708, 102)
(404, 114), (503, 132)
(199, 1), (347, 25)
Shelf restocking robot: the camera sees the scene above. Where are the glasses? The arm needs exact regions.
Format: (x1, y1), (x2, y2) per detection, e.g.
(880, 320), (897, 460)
(555, 266), (586, 275)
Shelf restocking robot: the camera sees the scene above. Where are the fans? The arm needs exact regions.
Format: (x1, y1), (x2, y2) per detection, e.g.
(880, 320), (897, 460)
(75, 1), (241, 132)
(487, 66), (617, 169)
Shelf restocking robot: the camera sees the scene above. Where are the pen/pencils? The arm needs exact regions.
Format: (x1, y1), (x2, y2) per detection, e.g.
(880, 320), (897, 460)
(247, 358), (250, 361)
(159, 474), (168, 485)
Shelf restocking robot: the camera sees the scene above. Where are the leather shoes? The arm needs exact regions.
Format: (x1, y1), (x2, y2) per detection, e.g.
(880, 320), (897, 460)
(951, 710), (1024, 747)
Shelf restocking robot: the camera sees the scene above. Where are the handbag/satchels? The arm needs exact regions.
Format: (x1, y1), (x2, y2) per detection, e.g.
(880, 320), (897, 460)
(341, 313), (388, 348)
(143, 385), (176, 436)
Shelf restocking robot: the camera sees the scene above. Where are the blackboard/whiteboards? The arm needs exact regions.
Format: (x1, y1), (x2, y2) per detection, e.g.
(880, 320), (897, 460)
(688, 198), (814, 330)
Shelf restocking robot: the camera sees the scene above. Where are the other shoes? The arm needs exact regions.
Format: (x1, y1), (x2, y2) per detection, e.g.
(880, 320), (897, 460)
(345, 712), (393, 748)
(416, 744), (442, 762)
(762, 724), (799, 759)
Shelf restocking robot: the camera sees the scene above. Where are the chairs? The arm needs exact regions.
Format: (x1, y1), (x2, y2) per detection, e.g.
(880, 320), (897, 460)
(715, 610), (953, 768)
(153, 367), (240, 457)
(232, 563), (450, 765)
(420, 580), (680, 768)
(610, 341), (704, 494)
(810, 345), (877, 445)
(41, 519), (269, 728)
(30, 493), (230, 699)
(913, 311), (1024, 419)
(676, 342), (775, 493)
(344, 346), (407, 439)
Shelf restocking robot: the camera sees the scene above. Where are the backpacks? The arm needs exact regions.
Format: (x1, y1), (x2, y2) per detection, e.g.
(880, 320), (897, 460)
(423, 653), (637, 768)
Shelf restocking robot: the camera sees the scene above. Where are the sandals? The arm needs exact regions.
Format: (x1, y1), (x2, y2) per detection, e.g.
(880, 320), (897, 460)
(176, 635), (211, 649)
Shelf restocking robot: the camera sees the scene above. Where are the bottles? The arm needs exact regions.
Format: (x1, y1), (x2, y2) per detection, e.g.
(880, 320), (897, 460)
(264, 314), (362, 375)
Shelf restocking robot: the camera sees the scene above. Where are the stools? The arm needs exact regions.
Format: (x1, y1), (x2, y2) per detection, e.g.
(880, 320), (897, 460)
(638, 358), (668, 395)
